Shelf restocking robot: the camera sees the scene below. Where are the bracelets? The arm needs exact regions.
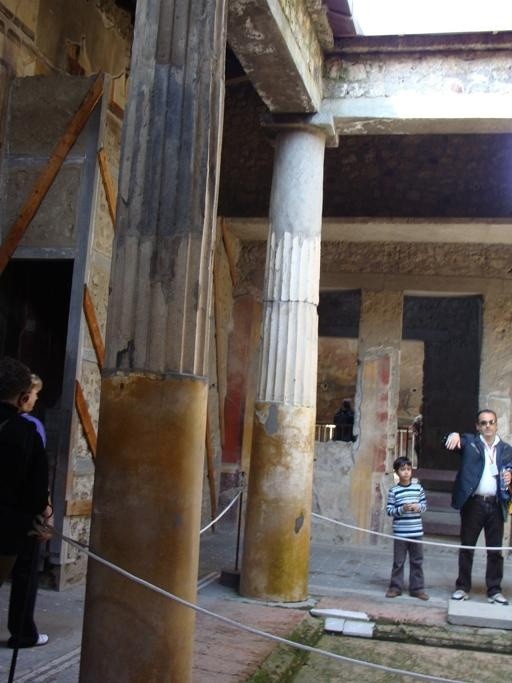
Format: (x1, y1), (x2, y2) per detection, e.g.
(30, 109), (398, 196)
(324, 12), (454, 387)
(48, 504), (53, 518)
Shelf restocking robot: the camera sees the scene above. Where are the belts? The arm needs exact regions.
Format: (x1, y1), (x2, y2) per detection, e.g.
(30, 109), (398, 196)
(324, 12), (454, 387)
(476, 495), (496, 502)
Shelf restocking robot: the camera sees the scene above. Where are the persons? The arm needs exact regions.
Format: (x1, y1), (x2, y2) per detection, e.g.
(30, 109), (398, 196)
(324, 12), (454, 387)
(444, 410), (511, 601)
(386, 456), (428, 598)
(19, 373), (47, 447)
(0, 358), (54, 644)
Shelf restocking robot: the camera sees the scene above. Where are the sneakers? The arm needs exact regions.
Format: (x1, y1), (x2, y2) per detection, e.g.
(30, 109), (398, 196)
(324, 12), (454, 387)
(36, 634), (49, 644)
(451, 590), (469, 602)
(487, 593), (508, 605)
(417, 593), (429, 600)
(386, 591), (400, 598)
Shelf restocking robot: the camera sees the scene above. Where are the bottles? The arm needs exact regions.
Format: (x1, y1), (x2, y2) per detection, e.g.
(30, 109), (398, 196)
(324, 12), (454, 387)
(499, 464), (510, 490)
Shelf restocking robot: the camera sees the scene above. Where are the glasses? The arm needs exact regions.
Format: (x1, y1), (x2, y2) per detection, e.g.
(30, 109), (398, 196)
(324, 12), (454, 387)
(479, 420), (495, 426)
